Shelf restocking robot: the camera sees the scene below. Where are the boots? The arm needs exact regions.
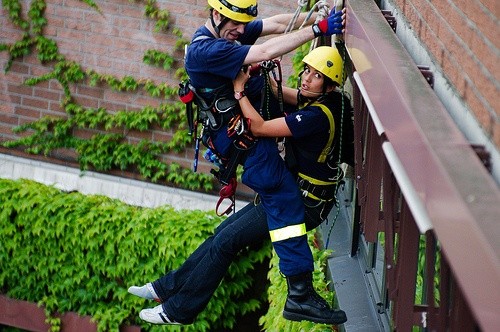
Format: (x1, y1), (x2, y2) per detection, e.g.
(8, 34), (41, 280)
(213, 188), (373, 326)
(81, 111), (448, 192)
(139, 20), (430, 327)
(282, 270), (348, 325)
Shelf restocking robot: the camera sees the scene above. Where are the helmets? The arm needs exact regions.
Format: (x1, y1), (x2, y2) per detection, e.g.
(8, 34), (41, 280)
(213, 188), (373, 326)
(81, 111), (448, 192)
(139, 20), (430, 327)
(301, 46), (347, 87)
(208, 0), (258, 23)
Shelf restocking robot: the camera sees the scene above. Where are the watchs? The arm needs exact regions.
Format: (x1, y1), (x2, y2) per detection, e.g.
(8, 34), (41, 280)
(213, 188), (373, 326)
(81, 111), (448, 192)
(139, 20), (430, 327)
(234, 91), (246, 100)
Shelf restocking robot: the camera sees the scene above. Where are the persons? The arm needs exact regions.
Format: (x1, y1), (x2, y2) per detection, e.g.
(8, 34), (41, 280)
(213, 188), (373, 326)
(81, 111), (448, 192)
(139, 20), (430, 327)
(127, 46), (352, 325)
(186, 0), (348, 325)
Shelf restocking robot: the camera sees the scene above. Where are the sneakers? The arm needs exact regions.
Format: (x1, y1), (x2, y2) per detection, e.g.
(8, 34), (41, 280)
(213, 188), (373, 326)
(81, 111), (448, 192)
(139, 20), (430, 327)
(128, 282), (161, 302)
(139, 304), (184, 324)
